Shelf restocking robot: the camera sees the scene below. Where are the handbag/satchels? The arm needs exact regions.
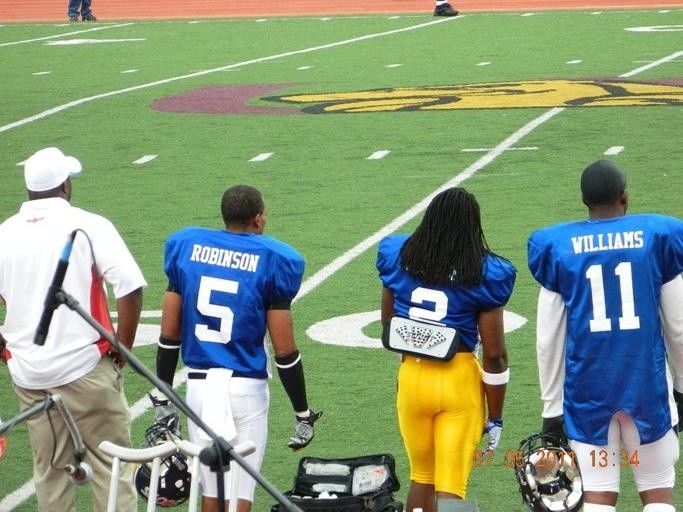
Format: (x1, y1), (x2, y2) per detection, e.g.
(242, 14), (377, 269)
(270, 453), (403, 512)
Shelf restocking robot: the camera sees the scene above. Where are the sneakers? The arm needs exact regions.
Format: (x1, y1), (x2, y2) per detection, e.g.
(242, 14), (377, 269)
(69, 14), (97, 22)
(433, 3), (458, 17)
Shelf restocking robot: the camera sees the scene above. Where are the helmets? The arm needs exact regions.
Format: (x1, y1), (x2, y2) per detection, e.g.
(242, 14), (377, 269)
(515, 431), (584, 512)
(133, 413), (191, 507)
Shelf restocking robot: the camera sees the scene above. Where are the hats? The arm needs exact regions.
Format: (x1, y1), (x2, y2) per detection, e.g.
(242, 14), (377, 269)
(24, 146), (82, 192)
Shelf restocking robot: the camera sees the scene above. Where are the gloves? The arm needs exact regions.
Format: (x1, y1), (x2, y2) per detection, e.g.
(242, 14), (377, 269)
(287, 409), (322, 452)
(479, 416), (502, 455)
(154, 391), (174, 417)
(673, 388), (683, 431)
(542, 414), (568, 448)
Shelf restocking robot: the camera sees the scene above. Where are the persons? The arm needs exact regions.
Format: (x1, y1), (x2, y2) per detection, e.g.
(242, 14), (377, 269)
(153, 184), (322, 512)
(433, 0), (459, 17)
(0, 148), (148, 512)
(376, 186), (510, 512)
(67, 0), (96, 24)
(527, 160), (683, 511)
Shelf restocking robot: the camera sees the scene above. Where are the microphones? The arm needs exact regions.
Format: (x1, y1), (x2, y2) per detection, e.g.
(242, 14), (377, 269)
(64, 463), (94, 486)
(34, 232), (77, 347)
